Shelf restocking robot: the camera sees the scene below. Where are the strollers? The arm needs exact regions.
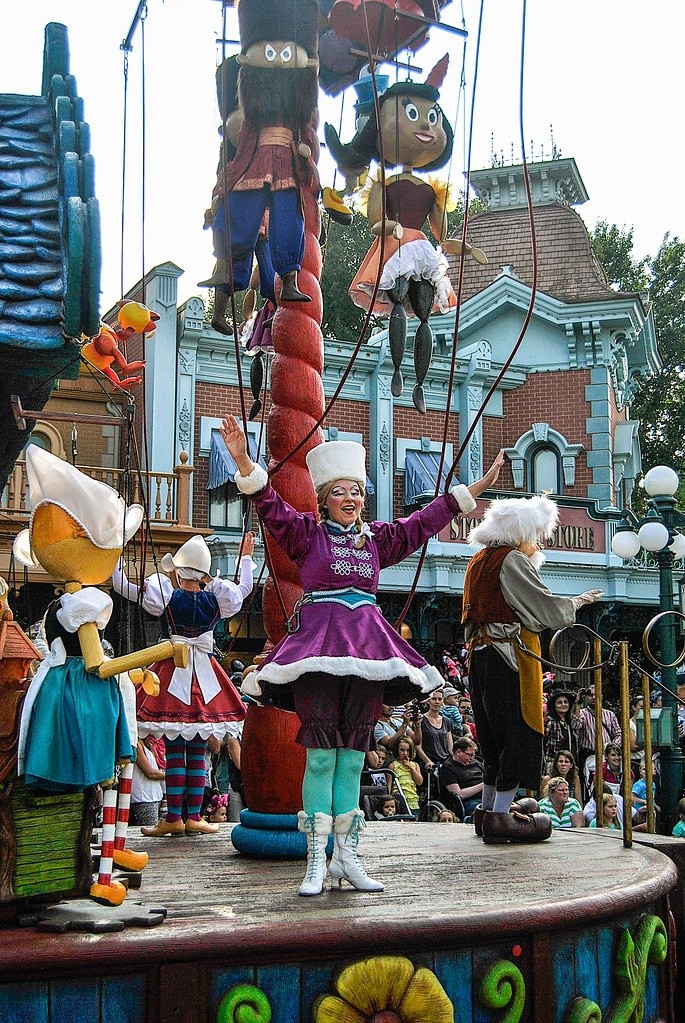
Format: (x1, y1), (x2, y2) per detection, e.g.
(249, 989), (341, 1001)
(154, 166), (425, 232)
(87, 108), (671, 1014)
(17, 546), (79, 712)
(358, 768), (417, 822)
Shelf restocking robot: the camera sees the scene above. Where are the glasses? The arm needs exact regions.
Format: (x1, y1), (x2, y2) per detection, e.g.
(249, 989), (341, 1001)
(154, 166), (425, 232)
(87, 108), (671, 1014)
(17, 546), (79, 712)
(462, 751), (476, 757)
(636, 695), (643, 699)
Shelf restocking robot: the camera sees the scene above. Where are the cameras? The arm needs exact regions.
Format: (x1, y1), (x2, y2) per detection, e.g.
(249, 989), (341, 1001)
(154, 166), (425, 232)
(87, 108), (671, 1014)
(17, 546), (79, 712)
(409, 705), (418, 721)
(580, 688), (591, 695)
(464, 708), (474, 715)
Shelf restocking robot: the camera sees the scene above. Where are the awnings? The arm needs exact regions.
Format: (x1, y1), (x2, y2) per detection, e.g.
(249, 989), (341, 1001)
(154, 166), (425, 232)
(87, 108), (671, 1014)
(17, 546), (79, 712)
(366, 474), (376, 496)
(403, 450), (460, 506)
(206, 431), (268, 489)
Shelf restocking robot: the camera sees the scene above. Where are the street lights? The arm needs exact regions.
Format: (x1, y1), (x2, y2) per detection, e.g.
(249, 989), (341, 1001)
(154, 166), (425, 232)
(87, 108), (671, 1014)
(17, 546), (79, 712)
(611, 465), (685, 838)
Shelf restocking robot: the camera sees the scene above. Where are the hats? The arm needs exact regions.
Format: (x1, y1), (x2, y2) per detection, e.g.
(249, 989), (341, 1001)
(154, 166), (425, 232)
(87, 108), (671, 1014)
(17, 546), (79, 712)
(306, 441), (368, 488)
(443, 687), (461, 699)
(161, 534), (222, 585)
(547, 688), (578, 713)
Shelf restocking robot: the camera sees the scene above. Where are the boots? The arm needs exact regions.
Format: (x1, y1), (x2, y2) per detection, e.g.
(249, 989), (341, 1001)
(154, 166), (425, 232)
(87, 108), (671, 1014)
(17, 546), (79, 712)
(326, 810), (385, 892)
(298, 810), (334, 895)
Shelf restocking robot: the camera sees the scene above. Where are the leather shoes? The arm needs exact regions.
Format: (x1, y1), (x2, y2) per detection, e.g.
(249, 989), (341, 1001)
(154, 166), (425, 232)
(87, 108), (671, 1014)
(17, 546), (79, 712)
(139, 818), (186, 837)
(185, 818), (220, 836)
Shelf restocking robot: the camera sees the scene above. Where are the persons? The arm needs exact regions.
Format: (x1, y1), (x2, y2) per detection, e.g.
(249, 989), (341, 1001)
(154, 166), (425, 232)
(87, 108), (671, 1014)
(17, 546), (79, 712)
(461, 496), (605, 844)
(11, 443), (189, 906)
(112, 531), (257, 837)
(196, 0), (390, 423)
(101, 638), (249, 825)
(360, 641), (685, 838)
(219, 413), (505, 896)
(325, 83), (488, 416)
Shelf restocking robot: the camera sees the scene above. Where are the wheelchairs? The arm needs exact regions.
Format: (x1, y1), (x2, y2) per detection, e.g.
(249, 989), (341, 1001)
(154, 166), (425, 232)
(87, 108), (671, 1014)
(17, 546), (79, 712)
(419, 764), (484, 822)
